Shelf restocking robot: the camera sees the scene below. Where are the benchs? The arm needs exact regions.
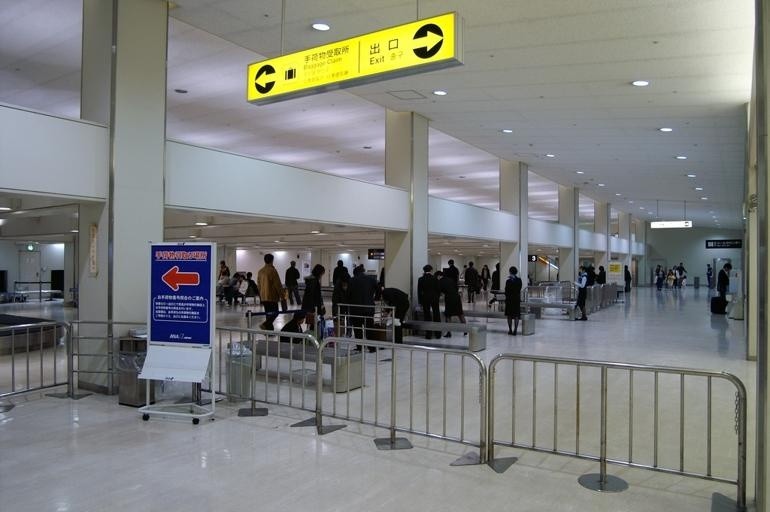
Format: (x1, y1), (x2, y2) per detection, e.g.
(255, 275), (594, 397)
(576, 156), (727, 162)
(377, 318), (488, 354)
(426, 311), (536, 335)
(280, 283), (337, 294)
(224, 336), (364, 392)
(519, 302), (576, 322)
(216, 282), (262, 307)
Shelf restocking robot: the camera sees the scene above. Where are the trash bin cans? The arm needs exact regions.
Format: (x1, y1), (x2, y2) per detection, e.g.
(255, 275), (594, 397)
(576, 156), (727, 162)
(119, 339), (156, 407)
(225, 351), (252, 403)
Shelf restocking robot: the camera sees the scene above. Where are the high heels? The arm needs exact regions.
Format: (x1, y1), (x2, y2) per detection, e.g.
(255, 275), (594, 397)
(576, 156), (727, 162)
(442, 332), (452, 338)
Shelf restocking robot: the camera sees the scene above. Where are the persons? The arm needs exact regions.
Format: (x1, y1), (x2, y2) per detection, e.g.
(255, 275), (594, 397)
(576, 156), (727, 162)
(705, 263), (713, 288)
(572, 265), (589, 322)
(716, 263), (732, 314)
(586, 257), (688, 293)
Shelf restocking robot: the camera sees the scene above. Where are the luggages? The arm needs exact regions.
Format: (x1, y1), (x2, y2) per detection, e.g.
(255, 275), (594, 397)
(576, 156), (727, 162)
(710, 292), (725, 315)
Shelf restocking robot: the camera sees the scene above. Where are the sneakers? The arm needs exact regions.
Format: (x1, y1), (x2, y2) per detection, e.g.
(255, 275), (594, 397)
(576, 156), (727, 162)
(508, 329), (518, 336)
(578, 314), (588, 321)
